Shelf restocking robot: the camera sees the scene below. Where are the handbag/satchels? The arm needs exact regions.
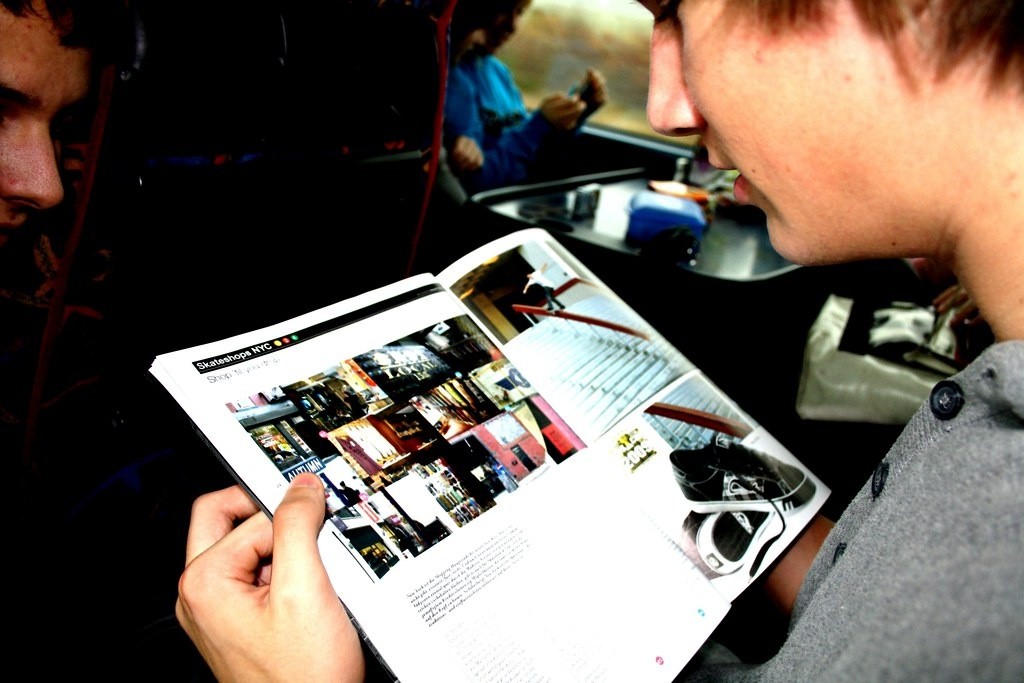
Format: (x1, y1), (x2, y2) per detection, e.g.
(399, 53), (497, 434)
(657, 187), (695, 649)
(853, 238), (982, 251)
(796, 293), (970, 424)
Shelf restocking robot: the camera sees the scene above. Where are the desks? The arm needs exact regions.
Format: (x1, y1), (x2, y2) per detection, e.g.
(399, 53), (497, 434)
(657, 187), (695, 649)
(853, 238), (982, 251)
(465, 163), (818, 306)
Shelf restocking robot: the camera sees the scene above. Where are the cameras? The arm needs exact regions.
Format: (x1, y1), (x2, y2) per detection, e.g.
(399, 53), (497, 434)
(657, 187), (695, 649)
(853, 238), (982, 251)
(573, 183), (601, 216)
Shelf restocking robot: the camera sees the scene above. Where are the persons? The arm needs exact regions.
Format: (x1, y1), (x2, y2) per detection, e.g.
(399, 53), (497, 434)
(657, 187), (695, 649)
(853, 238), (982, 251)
(177, 1), (1024, 682)
(414, 0), (606, 272)
(929, 283), (989, 329)
(0, 1), (141, 250)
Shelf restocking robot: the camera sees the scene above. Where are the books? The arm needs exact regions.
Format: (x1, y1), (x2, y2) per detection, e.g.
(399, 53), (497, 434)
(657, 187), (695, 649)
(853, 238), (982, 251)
(149, 226), (833, 683)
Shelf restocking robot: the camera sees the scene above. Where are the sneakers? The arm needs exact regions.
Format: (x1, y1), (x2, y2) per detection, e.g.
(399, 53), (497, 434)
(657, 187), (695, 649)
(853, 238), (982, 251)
(669, 437), (816, 515)
(682, 510), (774, 576)
